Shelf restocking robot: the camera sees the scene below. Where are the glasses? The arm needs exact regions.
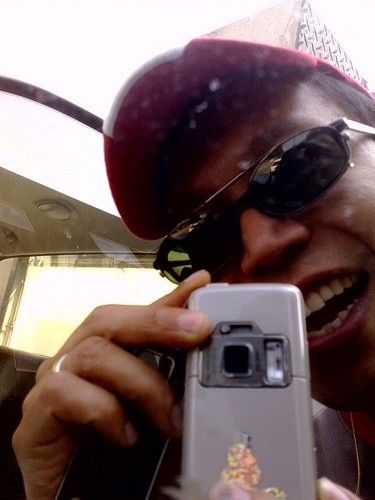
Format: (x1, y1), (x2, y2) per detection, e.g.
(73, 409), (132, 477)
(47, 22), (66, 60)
(154, 115), (375, 285)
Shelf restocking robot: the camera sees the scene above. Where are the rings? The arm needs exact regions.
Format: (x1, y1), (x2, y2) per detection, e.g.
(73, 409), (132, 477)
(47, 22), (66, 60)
(50, 354), (67, 373)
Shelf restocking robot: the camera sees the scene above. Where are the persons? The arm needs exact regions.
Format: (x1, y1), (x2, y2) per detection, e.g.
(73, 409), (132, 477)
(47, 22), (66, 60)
(9, 34), (375, 499)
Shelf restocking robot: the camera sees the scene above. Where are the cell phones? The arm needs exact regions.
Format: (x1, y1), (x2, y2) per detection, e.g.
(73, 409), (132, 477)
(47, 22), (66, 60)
(181, 282), (314, 500)
(53, 342), (186, 500)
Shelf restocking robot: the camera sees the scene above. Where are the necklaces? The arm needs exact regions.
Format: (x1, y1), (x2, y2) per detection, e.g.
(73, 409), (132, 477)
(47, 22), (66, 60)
(348, 412), (362, 498)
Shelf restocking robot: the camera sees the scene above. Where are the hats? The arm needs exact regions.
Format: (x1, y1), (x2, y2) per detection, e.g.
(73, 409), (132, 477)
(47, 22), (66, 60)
(103, 1), (373, 242)
